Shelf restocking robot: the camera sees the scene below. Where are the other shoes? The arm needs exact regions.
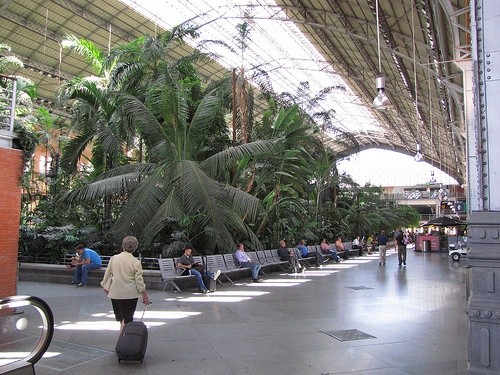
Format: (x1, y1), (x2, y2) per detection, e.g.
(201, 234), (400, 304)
(369, 252), (371, 254)
(70, 280), (80, 285)
(252, 276), (259, 280)
(199, 289), (209, 293)
(383, 260), (385, 265)
(379, 263), (381, 265)
(322, 258), (329, 263)
(77, 282), (87, 287)
(213, 270), (221, 281)
(403, 260), (406, 265)
(253, 279), (260, 283)
(359, 254), (363, 256)
(399, 263), (401, 266)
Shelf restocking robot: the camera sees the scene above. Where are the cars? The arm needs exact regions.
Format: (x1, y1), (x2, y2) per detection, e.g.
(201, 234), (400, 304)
(449, 236), (467, 262)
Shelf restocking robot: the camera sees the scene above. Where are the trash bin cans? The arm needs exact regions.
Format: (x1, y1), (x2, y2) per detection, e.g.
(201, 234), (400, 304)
(422, 239), (430, 252)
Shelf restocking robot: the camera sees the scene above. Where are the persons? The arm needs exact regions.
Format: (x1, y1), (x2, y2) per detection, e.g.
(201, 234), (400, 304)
(320, 238), (341, 264)
(234, 243), (261, 283)
(100, 236), (150, 331)
(378, 230), (389, 266)
(70, 245), (102, 287)
(352, 234), (378, 256)
(393, 227), (418, 246)
(296, 239), (329, 269)
(178, 247), (221, 292)
(277, 240), (306, 271)
(396, 229), (406, 266)
(335, 237), (352, 259)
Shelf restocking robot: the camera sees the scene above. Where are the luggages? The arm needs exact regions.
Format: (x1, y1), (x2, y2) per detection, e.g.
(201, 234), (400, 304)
(115, 301), (153, 364)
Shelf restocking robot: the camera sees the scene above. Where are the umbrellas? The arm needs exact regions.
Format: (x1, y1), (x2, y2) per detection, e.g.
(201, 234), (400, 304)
(421, 217), (468, 236)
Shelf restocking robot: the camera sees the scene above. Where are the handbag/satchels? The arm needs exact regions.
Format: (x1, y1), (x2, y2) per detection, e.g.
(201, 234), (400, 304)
(102, 255), (118, 292)
(193, 263), (205, 275)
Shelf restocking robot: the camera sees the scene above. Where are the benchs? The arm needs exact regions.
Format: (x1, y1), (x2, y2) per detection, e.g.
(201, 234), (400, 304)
(158, 241), (379, 294)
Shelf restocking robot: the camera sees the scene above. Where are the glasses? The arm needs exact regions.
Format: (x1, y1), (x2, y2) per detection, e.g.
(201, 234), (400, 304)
(76, 251), (80, 253)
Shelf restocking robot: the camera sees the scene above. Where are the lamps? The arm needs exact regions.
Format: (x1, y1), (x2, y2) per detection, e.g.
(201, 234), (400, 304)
(371, 0), (393, 109)
(407, 0), (457, 213)
